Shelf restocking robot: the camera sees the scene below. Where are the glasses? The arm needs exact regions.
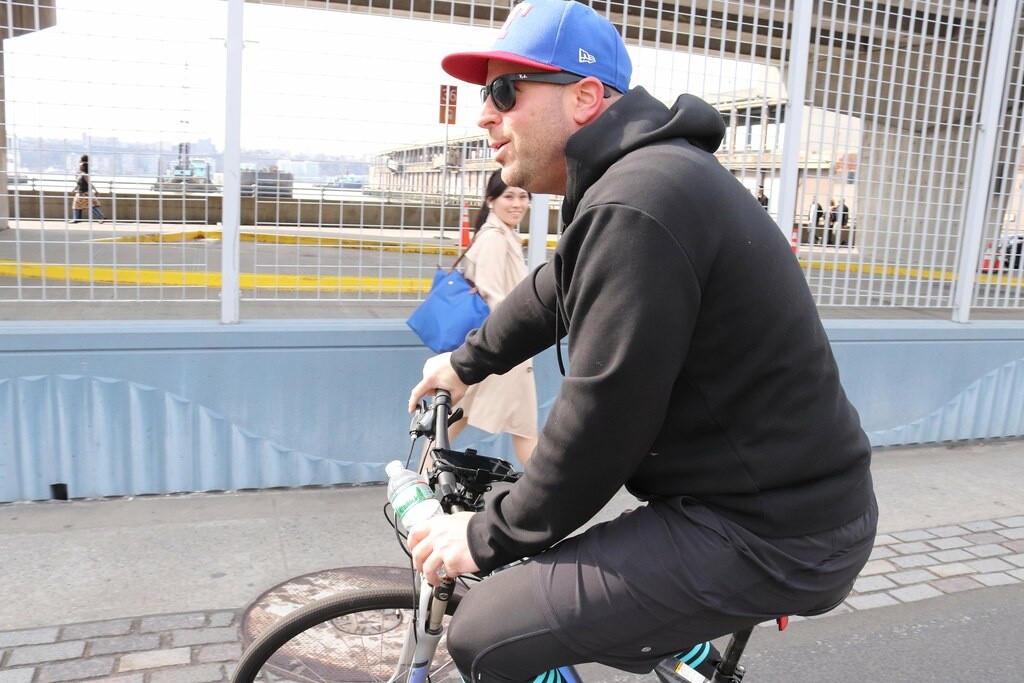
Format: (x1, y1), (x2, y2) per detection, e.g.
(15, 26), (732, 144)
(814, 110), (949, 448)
(480, 72), (613, 113)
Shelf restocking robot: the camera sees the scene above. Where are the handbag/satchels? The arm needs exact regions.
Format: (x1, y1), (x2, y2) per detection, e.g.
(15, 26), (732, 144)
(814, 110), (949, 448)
(405, 227), (493, 356)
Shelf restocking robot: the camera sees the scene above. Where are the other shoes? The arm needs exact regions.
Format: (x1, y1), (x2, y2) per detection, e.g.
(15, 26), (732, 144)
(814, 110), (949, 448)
(70, 220), (78, 223)
(99, 218), (106, 224)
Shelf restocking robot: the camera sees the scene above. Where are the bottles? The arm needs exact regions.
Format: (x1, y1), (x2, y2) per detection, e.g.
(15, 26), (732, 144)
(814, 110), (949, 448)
(385, 459), (446, 579)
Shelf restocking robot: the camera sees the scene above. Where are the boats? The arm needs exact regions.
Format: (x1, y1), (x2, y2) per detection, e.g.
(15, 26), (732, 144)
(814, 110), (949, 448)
(152, 142), (219, 193)
(240, 167), (294, 198)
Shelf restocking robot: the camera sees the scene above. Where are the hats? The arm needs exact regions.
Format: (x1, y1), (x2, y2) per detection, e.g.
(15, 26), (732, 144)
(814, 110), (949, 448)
(442, 0), (633, 93)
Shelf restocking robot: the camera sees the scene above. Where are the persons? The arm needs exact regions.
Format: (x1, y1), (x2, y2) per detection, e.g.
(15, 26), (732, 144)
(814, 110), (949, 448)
(408, 0), (881, 683)
(406, 165), (539, 478)
(808, 195), (848, 246)
(755, 185), (769, 211)
(69, 156), (106, 224)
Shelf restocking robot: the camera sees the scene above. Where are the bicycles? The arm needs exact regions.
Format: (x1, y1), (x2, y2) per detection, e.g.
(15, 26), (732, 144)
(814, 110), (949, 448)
(229, 387), (861, 683)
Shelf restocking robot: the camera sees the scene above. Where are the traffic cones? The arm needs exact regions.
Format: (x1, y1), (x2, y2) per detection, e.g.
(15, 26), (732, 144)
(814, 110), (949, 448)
(454, 203), (471, 246)
